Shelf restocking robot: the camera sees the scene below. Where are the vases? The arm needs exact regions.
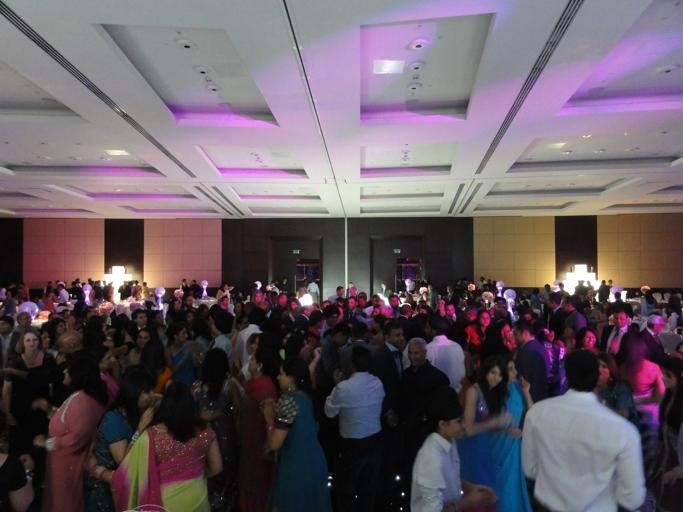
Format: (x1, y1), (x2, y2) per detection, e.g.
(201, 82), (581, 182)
(202, 286), (207, 296)
(157, 294), (163, 310)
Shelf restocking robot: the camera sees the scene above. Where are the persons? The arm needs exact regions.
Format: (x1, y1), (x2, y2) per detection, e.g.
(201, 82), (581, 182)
(0, 275), (683, 512)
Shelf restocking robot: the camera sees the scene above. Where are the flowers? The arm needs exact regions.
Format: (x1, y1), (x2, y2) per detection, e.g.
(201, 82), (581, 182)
(200, 280), (208, 287)
(155, 287), (165, 296)
(173, 289), (184, 298)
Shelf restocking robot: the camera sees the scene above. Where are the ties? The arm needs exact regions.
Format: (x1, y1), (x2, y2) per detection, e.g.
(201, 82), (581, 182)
(609, 330), (619, 353)
